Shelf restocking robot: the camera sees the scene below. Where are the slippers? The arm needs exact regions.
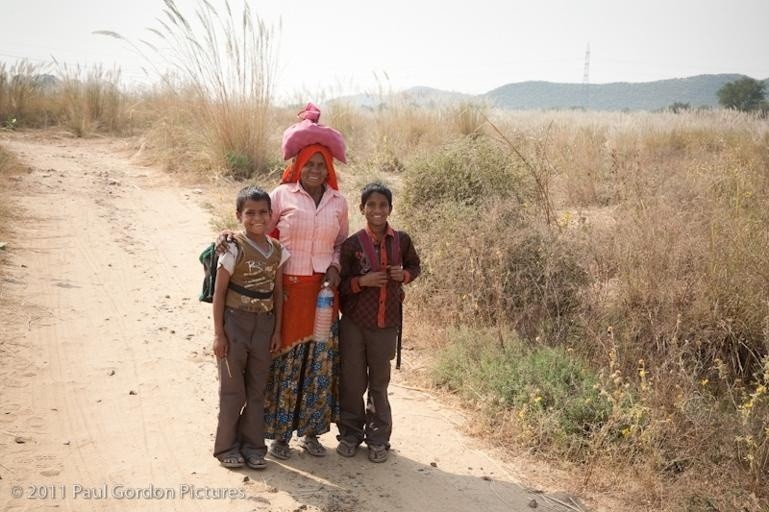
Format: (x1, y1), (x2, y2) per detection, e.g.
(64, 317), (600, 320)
(298, 435), (327, 457)
(218, 452), (244, 468)
(336, 436), (362, 458)
(368, 437), (389, 463)
(270, 440), (292, 460)
(246, 456), (268, 469)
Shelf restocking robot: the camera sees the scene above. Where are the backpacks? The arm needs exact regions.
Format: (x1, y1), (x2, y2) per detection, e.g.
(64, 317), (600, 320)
(198, 236), (242, 305)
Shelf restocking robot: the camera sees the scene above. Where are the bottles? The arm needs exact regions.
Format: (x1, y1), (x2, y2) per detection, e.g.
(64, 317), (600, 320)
(313, 280), (335, 345)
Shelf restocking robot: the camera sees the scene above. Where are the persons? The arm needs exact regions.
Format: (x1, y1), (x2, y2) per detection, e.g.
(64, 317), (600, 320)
(213, 184), (290, 471)
(337, 180), (421, 461)
(214, 142), (350, 460)
